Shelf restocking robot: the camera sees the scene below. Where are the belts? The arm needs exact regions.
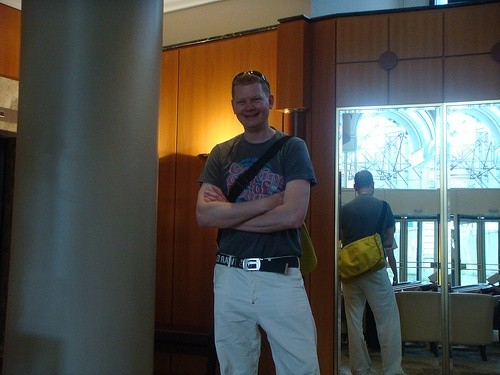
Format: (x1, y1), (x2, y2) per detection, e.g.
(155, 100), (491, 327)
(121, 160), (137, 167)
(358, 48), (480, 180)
(215, 253), (299, 274)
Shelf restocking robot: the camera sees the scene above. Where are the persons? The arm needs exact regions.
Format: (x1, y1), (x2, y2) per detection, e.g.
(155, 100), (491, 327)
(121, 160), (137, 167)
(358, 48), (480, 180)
(196, 70), (321, 375)
(363, 236), (400, 352)
(337, 170), (409, 375)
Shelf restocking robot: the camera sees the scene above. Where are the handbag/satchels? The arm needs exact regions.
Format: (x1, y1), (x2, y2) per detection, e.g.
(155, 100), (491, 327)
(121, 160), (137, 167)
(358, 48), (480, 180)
(298, 220), (318, 275)
(338, 232), (387, 281)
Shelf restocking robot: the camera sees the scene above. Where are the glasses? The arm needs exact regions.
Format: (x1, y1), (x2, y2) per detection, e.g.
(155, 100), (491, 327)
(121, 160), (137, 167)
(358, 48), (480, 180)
(231, 70), (269, 88)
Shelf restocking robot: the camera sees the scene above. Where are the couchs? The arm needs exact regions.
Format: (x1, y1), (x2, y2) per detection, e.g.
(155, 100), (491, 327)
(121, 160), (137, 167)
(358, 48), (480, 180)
(448, 283), (498, 362)
(392, 283), (442, 357)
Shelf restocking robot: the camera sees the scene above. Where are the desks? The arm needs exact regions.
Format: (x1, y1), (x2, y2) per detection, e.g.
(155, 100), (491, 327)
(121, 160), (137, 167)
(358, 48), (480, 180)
(393, 281), (432, 291)
(451, 285), (493, 293)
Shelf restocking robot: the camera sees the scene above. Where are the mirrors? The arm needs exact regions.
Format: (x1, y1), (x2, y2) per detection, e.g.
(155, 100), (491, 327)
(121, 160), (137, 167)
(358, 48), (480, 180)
(336, 99), (500, 375)
